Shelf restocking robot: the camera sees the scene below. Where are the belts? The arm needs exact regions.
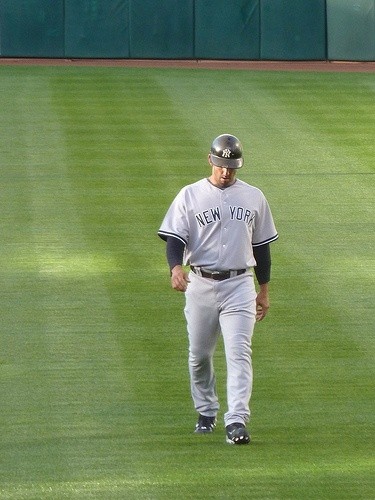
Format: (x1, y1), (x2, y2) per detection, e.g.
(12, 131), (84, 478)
(189, 266), (246, 280)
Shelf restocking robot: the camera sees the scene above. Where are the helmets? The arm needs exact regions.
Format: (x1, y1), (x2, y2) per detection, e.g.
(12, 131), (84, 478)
(210, 134), (244, 169)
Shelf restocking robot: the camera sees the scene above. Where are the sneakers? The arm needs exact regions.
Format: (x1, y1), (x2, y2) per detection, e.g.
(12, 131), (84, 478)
(226, 422), (250, 445)
(194, 414), (217, 433)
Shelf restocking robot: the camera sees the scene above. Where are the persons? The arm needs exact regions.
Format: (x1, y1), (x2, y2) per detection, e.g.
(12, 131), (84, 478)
(158, 134), (279, 445)
